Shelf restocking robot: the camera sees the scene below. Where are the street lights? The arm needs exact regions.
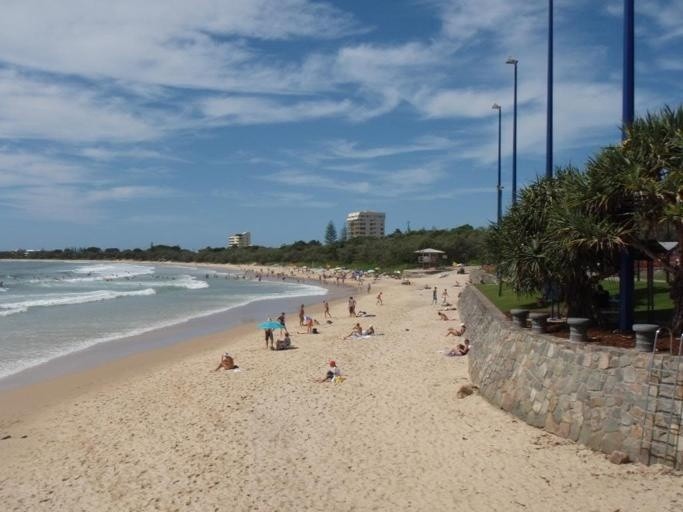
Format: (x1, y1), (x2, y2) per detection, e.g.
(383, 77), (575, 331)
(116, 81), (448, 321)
(491, 103), (503, 296)
(506, 59), (521, 300)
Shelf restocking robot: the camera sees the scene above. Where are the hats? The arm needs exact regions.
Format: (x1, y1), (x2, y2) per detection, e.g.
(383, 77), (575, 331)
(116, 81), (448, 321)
(328, 360), (336, 366)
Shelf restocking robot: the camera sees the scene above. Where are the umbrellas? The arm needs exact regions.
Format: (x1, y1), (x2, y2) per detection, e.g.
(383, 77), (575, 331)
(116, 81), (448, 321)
(413, 246), (447, 268)
(255, 321), (284, 335)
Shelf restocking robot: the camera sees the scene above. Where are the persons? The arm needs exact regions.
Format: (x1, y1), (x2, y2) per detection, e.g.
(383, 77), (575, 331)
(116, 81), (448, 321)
(214, 351), (233, 373)
(274, 332), (291, 350)
(264, 316), (273, 350)
(317, 360), (340, 384)
(421, 278), (467, 339)
(305, 317), (311, 336)
(346, 296), (357, 318)
(446, 338), (470, 356)
(374, 292), (383, 306)
(454, 261), (464, 273)
(298, 303), (304, 329)
(360, 324), (375, 336)
(354, 310), (367, 318)
(346, 323), (362, 336)
(203, 260), (381, 294)
(275, 311), (290, 335)
(322, 300), (331, 319)
